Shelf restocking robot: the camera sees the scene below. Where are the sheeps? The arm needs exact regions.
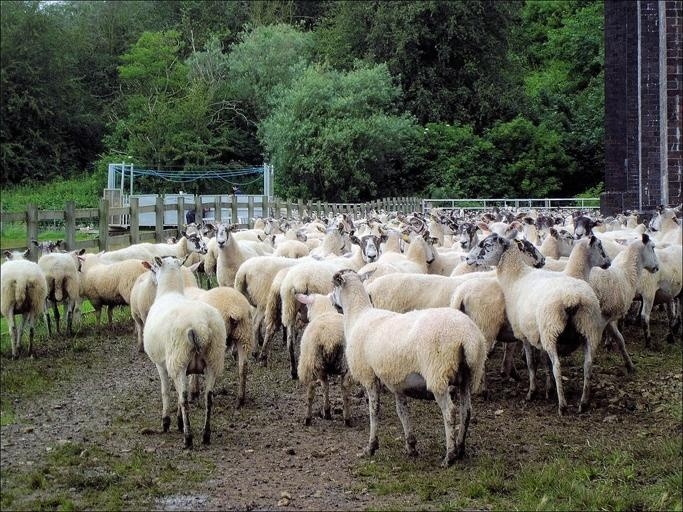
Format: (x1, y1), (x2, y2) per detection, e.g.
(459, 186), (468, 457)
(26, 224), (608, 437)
(0, 247), (49, 360)
(32, 238), (81, 340)
(70, 207), (683, 471)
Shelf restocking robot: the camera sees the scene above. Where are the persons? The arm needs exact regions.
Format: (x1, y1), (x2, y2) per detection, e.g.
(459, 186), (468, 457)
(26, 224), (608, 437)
(187, 208), (210, 223)
